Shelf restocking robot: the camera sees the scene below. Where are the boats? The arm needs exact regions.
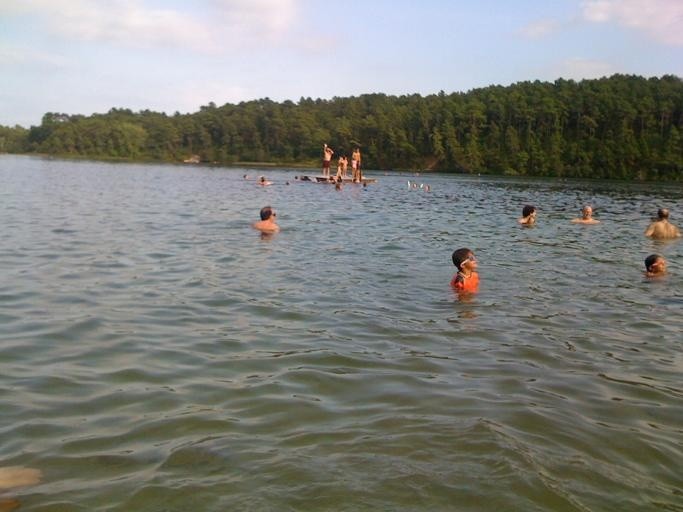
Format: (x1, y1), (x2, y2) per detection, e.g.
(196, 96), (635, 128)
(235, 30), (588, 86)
(300, 175), (377, 184)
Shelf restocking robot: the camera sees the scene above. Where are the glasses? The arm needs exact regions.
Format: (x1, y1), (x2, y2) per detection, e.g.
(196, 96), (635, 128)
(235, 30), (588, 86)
(461, 256), (475, 265)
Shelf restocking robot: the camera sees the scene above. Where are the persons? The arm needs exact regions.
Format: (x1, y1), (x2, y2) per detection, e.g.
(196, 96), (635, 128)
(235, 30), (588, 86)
(253, 205), (279, 231)
(451, 248), (480, 288)
(363, 182), (368, 186)
(322, 143), (333, 176)
(257, 176), (268, 186)
(243, 174), (248, 179)
(335, 184), (341, 191)
(336, 153), (348, 176)
(572, 205), (600, 222)
(517, 205), (536, 224)
(424, 185), (430, 191)
(412, 183), (417, 188)
(327, 177), (334, 184)
(350, 156), (357, 178)
(336, 177), (342, 184)
(645, 208), (679, 239)
(351, 147), (359, 166)
(642, 255), (666, 276)
